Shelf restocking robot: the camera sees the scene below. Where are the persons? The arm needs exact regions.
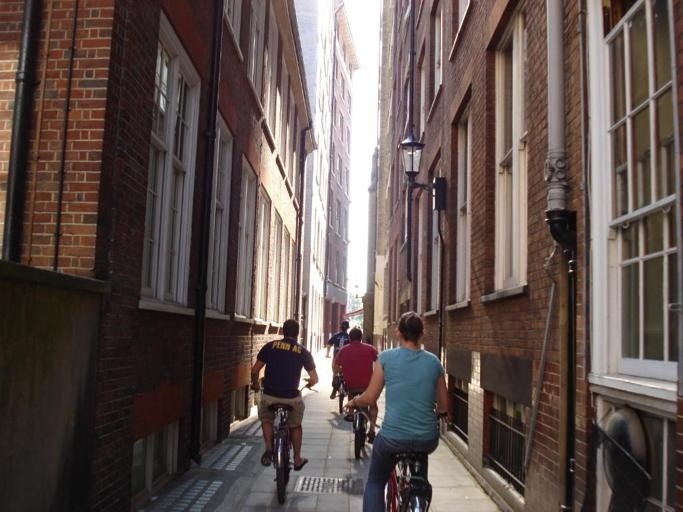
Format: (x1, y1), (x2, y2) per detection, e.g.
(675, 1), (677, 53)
(330, 327), (380, 442)
(249, 318), (317, 470)
(325, 320), (349, 398)
(342, 310), (450, 512)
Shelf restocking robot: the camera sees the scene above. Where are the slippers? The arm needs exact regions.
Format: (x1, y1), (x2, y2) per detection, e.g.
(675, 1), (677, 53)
(260, 448), (274, 467)
(293, 457), (308, 471)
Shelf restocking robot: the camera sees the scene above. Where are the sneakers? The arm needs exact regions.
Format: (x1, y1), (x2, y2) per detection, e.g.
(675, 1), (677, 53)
(367, 430), (376, 444)
(343, 415), (354, 422)
(330, 388), (339, 399)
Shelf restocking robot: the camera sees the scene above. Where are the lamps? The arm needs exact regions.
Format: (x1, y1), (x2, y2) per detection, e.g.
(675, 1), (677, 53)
(399, 127), (446, 213)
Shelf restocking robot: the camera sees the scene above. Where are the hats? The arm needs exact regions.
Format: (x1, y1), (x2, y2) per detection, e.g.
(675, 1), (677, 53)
(340, 321), (350, 329)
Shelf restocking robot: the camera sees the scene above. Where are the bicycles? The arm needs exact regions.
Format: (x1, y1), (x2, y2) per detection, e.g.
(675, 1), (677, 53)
(350, 391), (375, 460)
(325, 365), (347, 413)
(379, 410), (449, 512)
(246, 380), (319, 502)
